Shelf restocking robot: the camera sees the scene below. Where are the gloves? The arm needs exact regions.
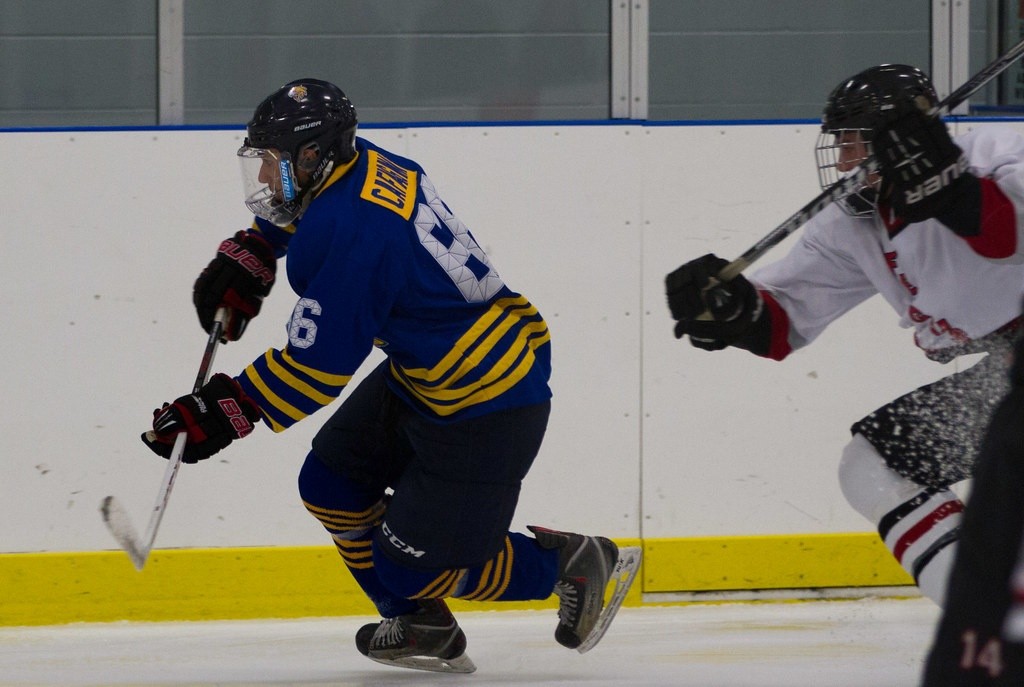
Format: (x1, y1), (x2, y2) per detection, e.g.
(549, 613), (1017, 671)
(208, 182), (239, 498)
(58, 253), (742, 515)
(865, 92), (980, 237)
(142, 374), (258, 465)
(193, 229), (277, 345)
(664, 254), (772, 358)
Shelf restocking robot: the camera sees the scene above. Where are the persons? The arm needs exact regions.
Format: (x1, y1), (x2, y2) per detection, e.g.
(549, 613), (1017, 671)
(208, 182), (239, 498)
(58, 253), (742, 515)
(140, 76), (642, 676)
(663, 63), (1024, 687)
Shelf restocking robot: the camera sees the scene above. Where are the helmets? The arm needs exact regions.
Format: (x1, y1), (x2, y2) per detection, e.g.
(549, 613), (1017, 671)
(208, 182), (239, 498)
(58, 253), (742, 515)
(815, 63), (964, 219)
(237, 78), (357, 227)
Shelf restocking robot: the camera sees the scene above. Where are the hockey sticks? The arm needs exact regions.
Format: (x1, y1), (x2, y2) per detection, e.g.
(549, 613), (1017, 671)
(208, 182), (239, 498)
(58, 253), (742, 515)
(98, 307), (224, 572)
(673, 40), (1024, 339)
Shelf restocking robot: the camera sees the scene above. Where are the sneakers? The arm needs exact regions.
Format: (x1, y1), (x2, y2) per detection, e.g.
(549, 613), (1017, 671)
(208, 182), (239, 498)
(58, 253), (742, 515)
(355, 598), (476, 674)
(527, 525), (641, 656)
